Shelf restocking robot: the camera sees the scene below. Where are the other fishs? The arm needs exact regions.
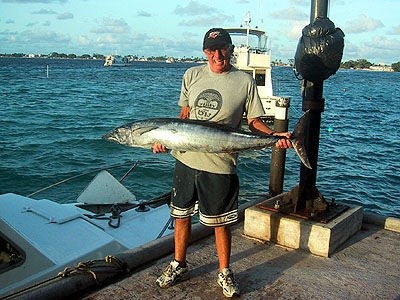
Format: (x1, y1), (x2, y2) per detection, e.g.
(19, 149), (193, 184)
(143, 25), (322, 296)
(101, 109), (312, 171)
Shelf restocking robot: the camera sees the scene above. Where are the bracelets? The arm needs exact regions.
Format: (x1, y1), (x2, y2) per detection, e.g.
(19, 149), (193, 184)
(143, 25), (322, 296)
(269, 131), (276, 135)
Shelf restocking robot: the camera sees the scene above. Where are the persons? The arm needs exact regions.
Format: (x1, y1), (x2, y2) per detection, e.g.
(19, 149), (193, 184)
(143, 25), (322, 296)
(153, 28), (291, 298)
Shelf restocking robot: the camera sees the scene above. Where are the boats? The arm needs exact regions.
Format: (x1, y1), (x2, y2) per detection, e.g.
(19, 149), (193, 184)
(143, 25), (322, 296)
(104, 54), (138, 67)
(220, 12), (288, 124)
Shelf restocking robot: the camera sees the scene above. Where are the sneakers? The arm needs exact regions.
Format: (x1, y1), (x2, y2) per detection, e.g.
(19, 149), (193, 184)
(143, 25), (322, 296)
(156, 259), (189, 289)
(216, 268), (240, 298)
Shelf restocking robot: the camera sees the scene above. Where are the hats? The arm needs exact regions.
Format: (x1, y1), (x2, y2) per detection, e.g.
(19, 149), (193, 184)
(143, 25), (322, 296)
(202, 27), (232, 49)
